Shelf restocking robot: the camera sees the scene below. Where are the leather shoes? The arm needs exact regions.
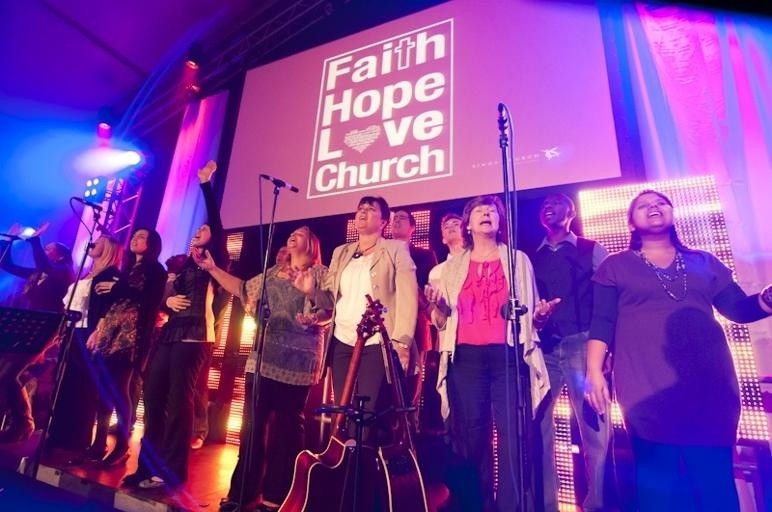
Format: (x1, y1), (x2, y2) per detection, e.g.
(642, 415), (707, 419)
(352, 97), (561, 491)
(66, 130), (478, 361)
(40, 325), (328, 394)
(191, 439), (203, 449)
(121, 473), (167, 489)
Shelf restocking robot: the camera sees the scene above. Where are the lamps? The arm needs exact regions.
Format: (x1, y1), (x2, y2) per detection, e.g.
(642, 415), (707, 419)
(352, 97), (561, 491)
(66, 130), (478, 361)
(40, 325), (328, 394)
(83, 176), (108, 204)
(185, 44), (204, 71)
(98, 106), (114, 130)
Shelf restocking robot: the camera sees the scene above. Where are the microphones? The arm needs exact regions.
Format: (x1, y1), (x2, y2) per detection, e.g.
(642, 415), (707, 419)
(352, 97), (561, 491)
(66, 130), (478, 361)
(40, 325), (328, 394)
(262, 174), (299, 193)
(498, 105), (510, 147)
(74, 196), (113, 215)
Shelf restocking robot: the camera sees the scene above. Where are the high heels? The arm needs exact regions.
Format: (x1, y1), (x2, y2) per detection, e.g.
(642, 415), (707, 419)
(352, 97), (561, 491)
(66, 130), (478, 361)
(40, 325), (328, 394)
(66, 444), (128, 469)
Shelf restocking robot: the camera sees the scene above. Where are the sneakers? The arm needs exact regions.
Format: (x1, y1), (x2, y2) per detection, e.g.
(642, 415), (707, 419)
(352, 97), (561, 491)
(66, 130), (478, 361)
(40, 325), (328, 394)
(219, 500), (278, 512)
(0, 417), (35, 443)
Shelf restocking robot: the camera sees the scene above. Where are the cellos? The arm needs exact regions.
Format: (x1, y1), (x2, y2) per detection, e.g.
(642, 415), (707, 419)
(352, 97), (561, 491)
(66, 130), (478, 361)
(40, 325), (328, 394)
(366, 294), (428, 512)
(277, 299), (386, 511)
